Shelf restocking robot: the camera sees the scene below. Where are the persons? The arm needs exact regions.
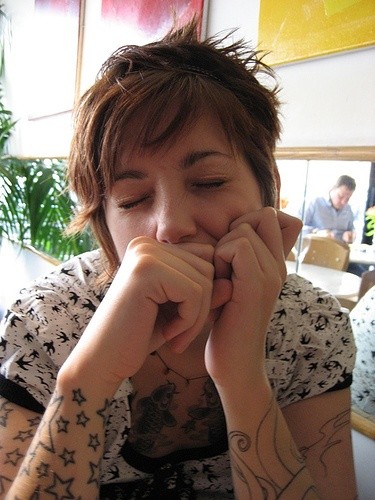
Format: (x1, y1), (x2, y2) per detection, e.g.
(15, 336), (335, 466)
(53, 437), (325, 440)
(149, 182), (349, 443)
(297, 175), (359, 245)
(0, 6), (359, 500)
(348, 284), (375, 412)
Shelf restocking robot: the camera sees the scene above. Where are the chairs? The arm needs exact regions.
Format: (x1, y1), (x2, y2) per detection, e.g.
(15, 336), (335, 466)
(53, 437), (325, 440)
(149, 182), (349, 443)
(337, 269), (375, 312)
(303, 237), (350, 273)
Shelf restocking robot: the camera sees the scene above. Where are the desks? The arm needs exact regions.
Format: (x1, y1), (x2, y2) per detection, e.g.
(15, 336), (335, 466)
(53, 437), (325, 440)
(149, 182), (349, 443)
(283, 259), (364, 300)
